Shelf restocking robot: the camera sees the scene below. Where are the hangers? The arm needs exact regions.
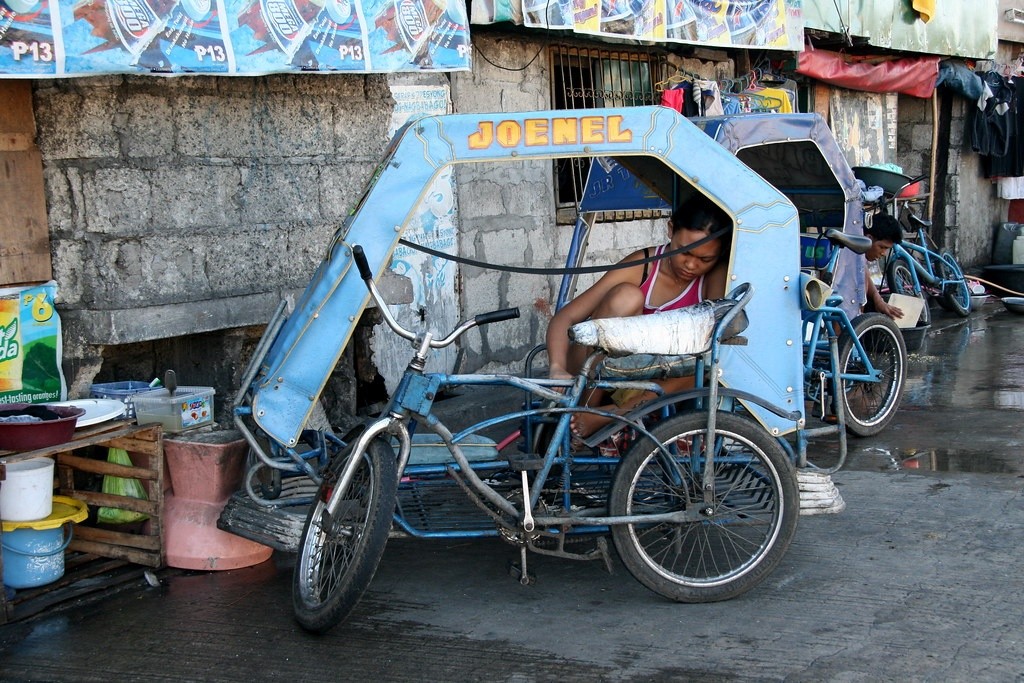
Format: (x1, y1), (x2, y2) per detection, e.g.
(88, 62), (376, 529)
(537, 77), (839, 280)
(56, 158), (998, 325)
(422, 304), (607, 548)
(654, 57), (795, 113)
(983, 53), (1024, 78)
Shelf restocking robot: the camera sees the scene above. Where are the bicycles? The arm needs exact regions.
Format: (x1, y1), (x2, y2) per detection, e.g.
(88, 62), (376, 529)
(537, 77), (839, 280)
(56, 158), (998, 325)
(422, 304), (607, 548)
(863, 172), (973, 324)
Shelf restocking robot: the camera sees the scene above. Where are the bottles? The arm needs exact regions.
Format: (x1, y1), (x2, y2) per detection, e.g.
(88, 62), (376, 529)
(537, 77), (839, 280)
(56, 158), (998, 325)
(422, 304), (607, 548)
(1012, 236), (1024, 264)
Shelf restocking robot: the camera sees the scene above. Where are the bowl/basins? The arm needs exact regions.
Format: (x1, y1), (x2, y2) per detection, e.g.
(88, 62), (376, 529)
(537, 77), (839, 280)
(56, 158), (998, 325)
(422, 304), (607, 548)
(1001, 297), (1024, 312)
(970, 294), (990, 311)
(983, 264), (1024, 297)
(899, 176), (919, 197)
(852, 166), (914, 197)
(0, 403), (86, 450)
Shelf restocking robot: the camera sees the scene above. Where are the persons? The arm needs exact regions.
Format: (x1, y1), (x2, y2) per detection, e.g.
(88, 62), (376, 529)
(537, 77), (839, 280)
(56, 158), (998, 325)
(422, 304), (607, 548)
(546, 197), (733, 437)
(811, 215), (904, 425)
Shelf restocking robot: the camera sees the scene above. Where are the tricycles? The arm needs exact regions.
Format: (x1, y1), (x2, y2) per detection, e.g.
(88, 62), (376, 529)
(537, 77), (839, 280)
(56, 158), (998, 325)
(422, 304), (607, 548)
(223, 106), (908, 632)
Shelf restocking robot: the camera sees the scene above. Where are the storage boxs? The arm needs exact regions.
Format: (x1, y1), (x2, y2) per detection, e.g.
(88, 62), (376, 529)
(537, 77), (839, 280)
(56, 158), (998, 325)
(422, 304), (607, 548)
(91, 380), (163, 422)
(133, 385), (216, 433)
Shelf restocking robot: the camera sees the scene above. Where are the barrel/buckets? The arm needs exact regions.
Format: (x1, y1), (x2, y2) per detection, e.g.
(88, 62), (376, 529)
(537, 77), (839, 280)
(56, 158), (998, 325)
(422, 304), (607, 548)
(0, 522), (73, 589)
(0, 457), (55, 523)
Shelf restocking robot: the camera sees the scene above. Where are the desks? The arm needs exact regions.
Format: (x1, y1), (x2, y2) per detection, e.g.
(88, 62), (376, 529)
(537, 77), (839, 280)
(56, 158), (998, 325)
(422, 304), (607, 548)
(0, 418), (166, 570)
